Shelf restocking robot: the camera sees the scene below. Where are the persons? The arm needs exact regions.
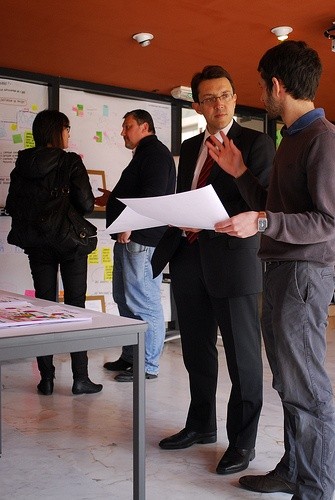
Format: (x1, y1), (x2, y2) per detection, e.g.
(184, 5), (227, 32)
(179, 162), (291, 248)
(94, 109), (176, 382)
(5, 108), (103, 394)
(150, 65), (276, 475)
(205, 40), (335, 500)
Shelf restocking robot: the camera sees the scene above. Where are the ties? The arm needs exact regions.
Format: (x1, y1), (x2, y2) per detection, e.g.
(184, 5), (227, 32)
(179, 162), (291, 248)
(185, 135), (215, 241)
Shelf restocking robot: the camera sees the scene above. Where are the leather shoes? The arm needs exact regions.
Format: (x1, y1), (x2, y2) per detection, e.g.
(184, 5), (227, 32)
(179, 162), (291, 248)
(238, 471), (294, 494)
(103, 357), (135, 371)
(159, 428), (217, 449)
(216, 448), (255, 475)
(36, 380), (54, 395)
(72, 376), (103, 394)
(114, 369), (157, 382)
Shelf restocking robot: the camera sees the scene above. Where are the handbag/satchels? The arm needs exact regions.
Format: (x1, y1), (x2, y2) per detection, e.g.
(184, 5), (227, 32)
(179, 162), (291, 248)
(41, 152), (89, 265)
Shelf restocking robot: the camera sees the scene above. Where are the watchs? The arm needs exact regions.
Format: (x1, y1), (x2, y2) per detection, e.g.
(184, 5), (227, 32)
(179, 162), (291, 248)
(258, 211), (268, 233)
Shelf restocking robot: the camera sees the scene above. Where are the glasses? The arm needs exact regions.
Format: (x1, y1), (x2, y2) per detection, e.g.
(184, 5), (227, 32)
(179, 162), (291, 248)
(199, 92), (235, 105)
(63, 124), (70, 132)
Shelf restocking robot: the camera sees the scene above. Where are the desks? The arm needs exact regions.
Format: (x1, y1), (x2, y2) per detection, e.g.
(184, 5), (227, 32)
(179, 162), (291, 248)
(0, 289), (148, 500)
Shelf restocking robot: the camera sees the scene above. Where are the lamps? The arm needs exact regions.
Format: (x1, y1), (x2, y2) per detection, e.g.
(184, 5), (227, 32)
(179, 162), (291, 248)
(270, 26), (293, 42)
(169, 86), (195, 103)
(133, 32), (154, 47)
